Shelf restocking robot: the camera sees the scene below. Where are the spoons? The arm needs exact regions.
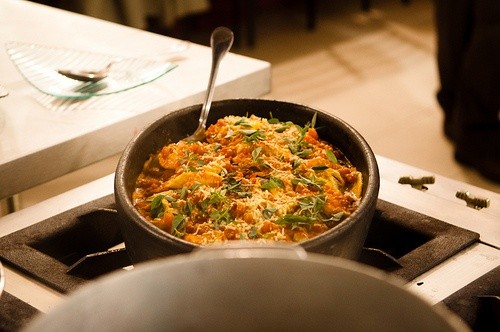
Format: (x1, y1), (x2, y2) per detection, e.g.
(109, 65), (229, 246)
(57, 54), (187, 83)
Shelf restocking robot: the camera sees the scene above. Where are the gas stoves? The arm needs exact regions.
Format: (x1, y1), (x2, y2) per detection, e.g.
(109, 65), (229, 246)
(0, 152), (500, 332)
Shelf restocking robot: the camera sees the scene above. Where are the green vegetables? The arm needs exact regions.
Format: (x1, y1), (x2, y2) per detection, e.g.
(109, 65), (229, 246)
(147, 112), (345, 241)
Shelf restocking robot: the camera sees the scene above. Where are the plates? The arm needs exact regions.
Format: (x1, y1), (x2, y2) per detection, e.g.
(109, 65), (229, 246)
(7, 40), (177, 99)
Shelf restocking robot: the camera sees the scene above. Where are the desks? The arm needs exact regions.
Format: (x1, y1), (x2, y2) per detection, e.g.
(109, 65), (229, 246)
(0, 0), (271, 217)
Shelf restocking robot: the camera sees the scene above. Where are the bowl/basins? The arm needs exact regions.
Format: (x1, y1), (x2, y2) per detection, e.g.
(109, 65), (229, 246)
(114, 98), (380, 264)
(19, 240), (468, 331)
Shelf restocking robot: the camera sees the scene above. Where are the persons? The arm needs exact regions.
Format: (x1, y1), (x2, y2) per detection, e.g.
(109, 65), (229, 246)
(432, 0), (500, 186)
